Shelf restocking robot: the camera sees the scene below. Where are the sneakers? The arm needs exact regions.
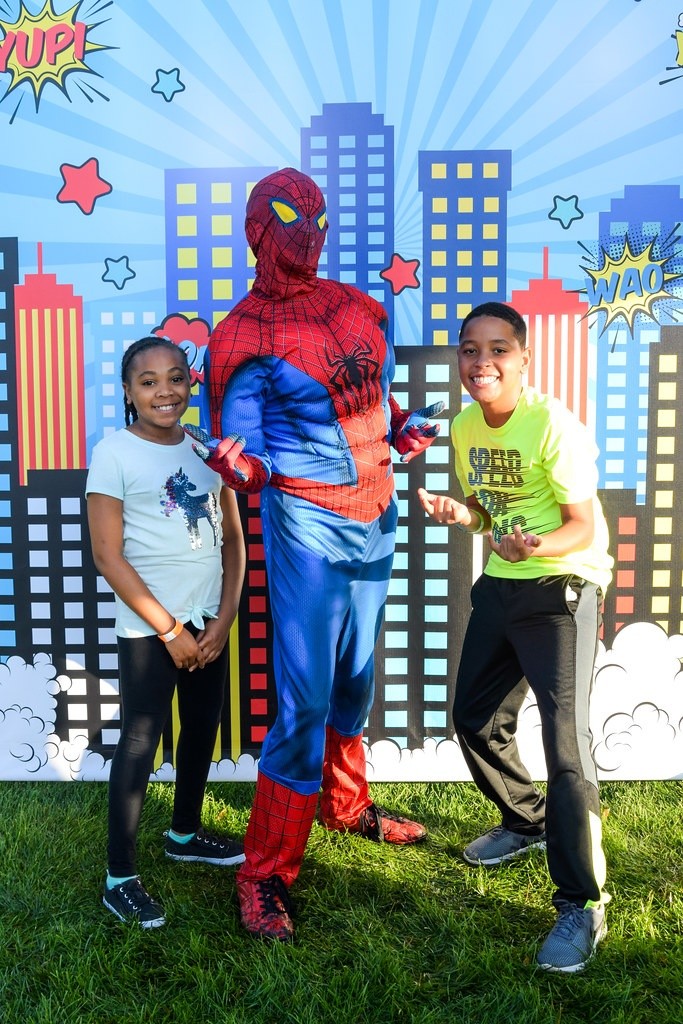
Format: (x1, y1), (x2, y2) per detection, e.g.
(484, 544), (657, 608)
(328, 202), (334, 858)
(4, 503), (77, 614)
(164, 826), (246, 866)
(535, 899), (608, 972)
(103, 875), (167, 928)
(238, 877), (297, 937)
(463, 822), (547, 866)
(341, 800), (428, 847)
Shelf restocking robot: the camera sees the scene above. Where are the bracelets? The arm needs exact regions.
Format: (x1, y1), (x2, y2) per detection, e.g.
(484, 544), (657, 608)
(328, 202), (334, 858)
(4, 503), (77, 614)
(454, 509), (484, 534)
(158, 618), (183, 643)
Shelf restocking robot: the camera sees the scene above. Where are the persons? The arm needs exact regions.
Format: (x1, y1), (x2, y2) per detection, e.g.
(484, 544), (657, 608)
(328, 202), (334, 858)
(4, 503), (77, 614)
(183, 167), (444, 939)
(416, 302), (612, 973)
(84, 337), (248, 927)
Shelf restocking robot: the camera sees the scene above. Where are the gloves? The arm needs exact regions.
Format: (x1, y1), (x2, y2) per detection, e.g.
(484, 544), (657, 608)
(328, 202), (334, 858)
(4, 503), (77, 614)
(183, 424), (268, 496)
(392, 402), (446, 463)
